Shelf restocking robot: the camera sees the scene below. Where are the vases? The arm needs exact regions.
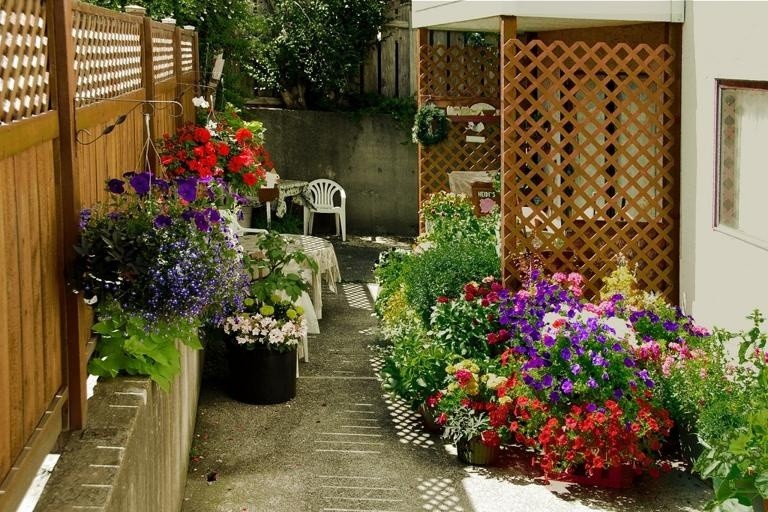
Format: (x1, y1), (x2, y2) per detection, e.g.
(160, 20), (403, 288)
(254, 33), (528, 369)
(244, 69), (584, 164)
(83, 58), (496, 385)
(217, 342), (299, 407)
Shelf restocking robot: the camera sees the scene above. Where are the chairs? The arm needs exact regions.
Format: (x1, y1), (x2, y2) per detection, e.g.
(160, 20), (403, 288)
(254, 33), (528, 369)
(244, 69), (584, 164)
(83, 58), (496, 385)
(300, 178), (348, 242)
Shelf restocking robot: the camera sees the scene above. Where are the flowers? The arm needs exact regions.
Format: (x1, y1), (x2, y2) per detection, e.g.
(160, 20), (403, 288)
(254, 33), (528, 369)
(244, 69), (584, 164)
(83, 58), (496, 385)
(153, 94), (273, 201)
(369, 188), (767, 511)
(61, 172), (250, 381)
(219, 291), (308, 355)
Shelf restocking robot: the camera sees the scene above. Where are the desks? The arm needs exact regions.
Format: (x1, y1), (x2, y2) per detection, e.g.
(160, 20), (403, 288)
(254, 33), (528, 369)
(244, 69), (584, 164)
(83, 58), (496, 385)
(236, 233), (333, 320)
(224, 234), (342, 321)
